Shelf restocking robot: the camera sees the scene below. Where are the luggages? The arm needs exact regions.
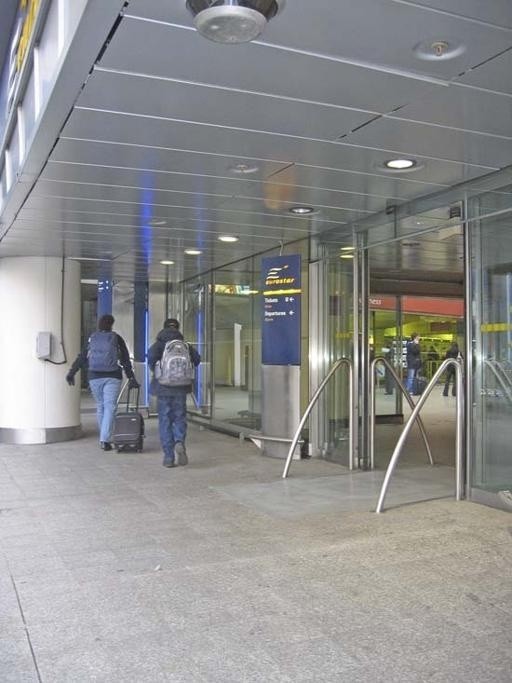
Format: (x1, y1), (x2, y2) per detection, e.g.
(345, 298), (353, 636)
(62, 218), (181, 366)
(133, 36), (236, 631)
(112, 385), (144, 452)
(413, 379), (425, 396)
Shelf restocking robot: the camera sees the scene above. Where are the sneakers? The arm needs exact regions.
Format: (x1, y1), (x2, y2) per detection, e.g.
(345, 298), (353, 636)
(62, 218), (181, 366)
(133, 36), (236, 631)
(164, 442), (187, 467)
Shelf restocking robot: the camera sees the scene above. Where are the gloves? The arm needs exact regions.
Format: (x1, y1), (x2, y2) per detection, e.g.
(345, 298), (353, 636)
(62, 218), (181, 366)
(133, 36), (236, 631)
(129, 377), (138, 389)
(66, 374), (74, 385)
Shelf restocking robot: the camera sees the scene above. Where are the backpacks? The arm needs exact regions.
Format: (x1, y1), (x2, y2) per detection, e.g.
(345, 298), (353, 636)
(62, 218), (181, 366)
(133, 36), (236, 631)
(89, 331), (120, 372)
(154, 339), (195, 386)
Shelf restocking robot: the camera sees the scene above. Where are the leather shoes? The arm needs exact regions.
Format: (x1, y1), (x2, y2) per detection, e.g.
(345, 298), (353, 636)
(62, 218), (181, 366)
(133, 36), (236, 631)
(101, 442), (111, 451)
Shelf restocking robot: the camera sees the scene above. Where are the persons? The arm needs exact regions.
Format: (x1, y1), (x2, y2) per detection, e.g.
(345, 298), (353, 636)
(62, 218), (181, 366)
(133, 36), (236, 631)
(442, 342), (464, 396)
(383, 338), (395, 395)
(147, 319), (201, 468)
(66, 314), (139, 451)
(406, 332), (422, 396)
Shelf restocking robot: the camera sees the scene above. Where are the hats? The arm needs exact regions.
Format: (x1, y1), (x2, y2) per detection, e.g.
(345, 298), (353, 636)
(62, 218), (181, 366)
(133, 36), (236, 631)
(164, 319), (179, 328)
